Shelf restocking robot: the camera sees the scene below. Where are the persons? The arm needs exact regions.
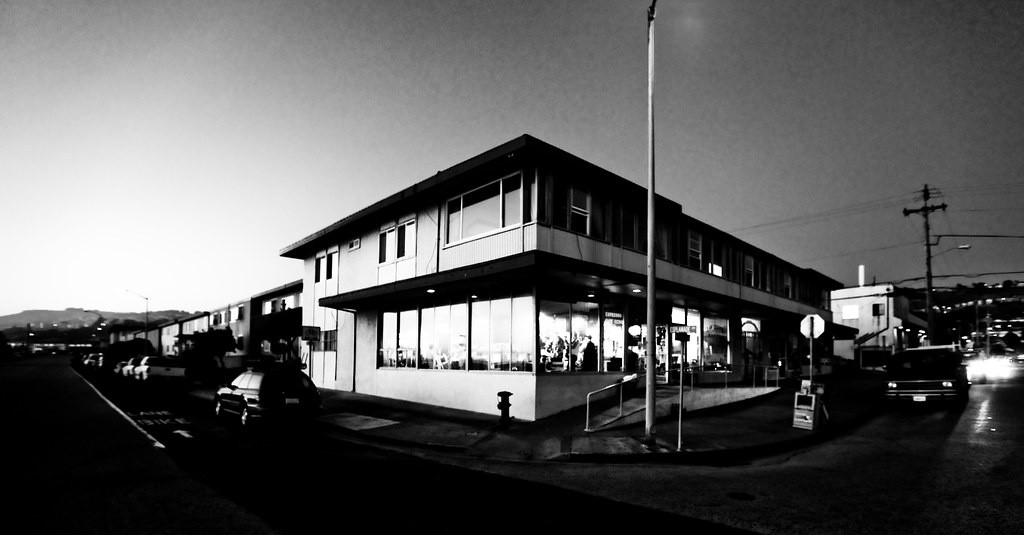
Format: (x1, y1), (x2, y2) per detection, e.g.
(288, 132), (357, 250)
(742, 347), (778, 386)
(1003, 327), (1021, 350)
(540, 332), (596, 373)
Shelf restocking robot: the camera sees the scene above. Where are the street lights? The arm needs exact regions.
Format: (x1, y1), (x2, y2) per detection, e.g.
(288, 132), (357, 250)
(924, 242), (972, 347)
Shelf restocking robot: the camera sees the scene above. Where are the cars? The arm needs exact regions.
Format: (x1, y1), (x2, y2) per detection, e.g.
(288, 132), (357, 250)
(81, 352), (187, 383)
(882, 346), (989, 415)
(212, 359), (325, 430)
